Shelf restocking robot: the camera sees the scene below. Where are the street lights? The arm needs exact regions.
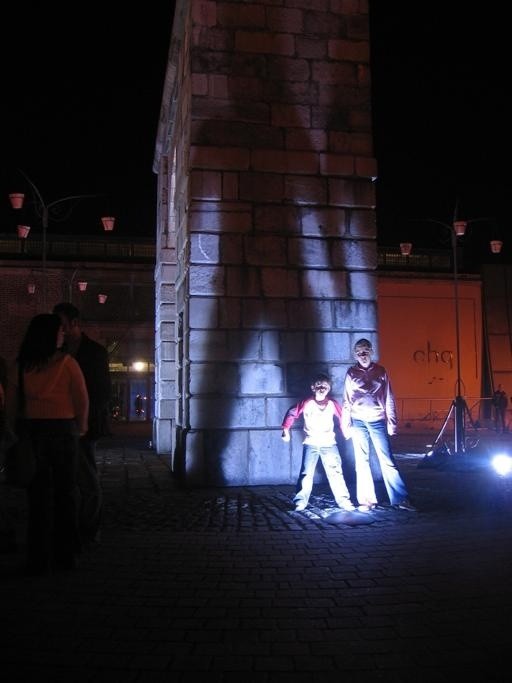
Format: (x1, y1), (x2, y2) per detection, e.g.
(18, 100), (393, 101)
(6, 166), (120, 317)
(386, 212), (512, 472)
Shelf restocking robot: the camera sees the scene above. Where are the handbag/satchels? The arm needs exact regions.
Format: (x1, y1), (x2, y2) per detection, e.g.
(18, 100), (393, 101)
(6, 417), (33, 489)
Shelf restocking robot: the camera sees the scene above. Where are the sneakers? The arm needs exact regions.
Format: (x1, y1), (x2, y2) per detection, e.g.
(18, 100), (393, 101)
(358, 503), (376, 512)
(399, 495), (422, 512)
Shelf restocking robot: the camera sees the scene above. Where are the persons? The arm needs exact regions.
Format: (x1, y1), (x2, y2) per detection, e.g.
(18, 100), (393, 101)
(6, 314), (90, 576)
(492, 384), (508, 433)
(54, 303), (112, 545)
(341, 339), (423, 511)
(281, 374), (355, 511)
